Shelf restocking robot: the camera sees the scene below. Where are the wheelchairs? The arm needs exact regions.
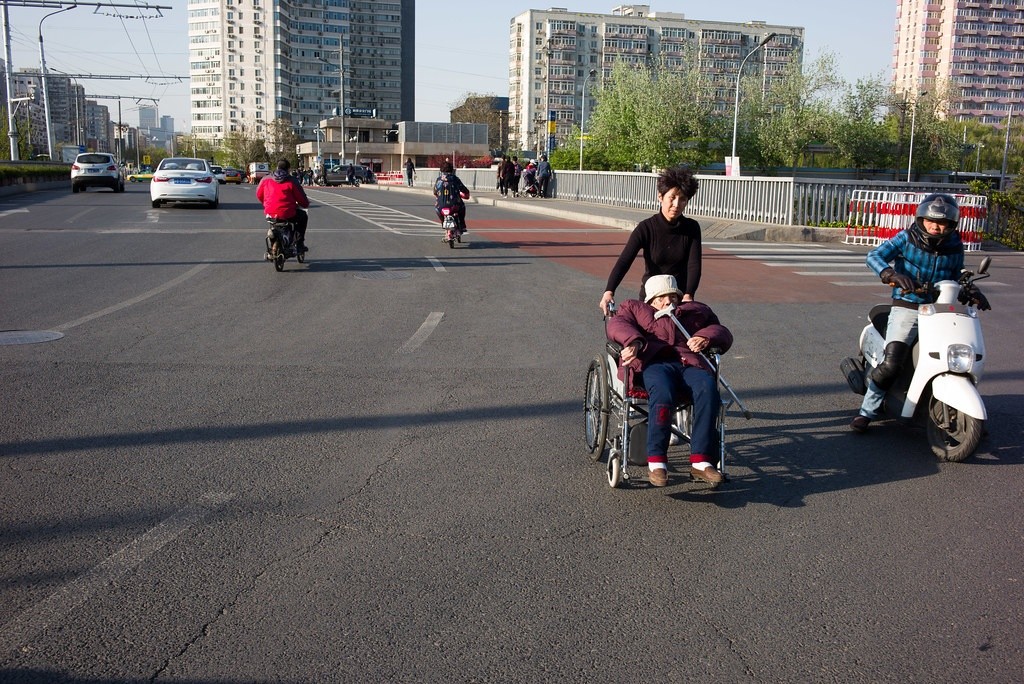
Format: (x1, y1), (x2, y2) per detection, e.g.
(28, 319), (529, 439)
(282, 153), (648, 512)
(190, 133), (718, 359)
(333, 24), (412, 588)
(583, 302), (727, 488)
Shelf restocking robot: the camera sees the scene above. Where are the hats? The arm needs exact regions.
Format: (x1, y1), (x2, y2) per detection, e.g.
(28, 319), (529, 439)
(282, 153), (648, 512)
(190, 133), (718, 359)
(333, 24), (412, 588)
(643, 275), (683, 304)
(530, 159), (536, 164)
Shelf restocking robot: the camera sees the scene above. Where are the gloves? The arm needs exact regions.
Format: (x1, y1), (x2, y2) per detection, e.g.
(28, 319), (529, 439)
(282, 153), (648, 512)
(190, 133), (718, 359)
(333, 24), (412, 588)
(879, 268), (920, 293)
(964, 285), (992, 312)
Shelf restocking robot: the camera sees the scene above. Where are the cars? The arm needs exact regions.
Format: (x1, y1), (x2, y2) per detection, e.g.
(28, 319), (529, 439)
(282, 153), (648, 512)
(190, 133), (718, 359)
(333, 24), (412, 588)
(314, 164), (375, 187)
(149, 157), (220, 209)
(71, 151), (125, 193)
(223, 167), (243, 185)
(210, 164), (227, 185)
(238, 170), (249, 183)
(127, 169), (155, 183)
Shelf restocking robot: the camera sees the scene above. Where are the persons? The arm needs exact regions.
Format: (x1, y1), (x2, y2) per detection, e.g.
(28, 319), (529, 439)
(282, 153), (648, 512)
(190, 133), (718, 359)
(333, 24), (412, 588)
(433, 162), (469, 231)
(606, 275), (733, 486)
(404, 158), (415, 187)
(600, 167), (703, 446)
(497, 154), (551, 198)
(445, 157), (450, 162)
(346, 163), (354, 187)
(256, 160), (309, 253)
(292, 164), (326, 186)
(849, 193), (991, 432)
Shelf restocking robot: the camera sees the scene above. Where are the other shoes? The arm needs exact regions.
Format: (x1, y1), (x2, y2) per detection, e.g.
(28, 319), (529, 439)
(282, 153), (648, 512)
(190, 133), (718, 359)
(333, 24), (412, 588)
(647, 467), (668, 487)
(851, 416), (870, 432)
(691, 466), (722, 482)
(462, 228), (467, 232)
(500, 193), (545, 199)
(298, 247), (308, 253)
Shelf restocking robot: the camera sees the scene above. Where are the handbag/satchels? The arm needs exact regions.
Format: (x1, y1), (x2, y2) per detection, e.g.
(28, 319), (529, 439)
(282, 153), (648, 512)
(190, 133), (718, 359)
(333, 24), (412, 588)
(414, 176), (418, 180)
(496, 180), (500, 189)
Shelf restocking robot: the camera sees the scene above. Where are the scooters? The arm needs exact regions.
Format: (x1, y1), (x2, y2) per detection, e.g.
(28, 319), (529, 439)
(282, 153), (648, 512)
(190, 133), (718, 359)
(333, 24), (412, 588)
(440, 192), (470, 249)
(264, 202), (305, 272)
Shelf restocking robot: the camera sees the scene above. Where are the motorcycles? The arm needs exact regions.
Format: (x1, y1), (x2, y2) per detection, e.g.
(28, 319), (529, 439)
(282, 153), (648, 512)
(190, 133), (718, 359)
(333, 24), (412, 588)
(841, 256), (992, 463)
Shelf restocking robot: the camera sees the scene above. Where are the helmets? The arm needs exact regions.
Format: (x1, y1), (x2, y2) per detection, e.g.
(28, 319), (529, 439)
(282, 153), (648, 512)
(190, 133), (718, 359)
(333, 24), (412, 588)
(915, 194), (960, 246)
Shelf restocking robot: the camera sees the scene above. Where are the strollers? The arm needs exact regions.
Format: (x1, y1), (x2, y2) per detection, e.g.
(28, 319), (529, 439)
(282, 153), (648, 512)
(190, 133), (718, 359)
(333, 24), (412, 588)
(519, 168), (539, 198)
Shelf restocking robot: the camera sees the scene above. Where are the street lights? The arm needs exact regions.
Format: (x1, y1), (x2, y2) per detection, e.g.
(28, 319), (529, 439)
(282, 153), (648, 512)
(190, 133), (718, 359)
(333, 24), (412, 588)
(39, 5), (77, 161)
(579, 69), (595, 171)
(730, 32), (777, 177)
(907, 90), (928, 182)
(317, 57), (345, 165)
(49, 67), (80, 146)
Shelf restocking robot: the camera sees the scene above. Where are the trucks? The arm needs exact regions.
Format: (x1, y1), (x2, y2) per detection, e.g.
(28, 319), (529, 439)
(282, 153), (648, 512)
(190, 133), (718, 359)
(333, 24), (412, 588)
(247, 162), (269, 185)
